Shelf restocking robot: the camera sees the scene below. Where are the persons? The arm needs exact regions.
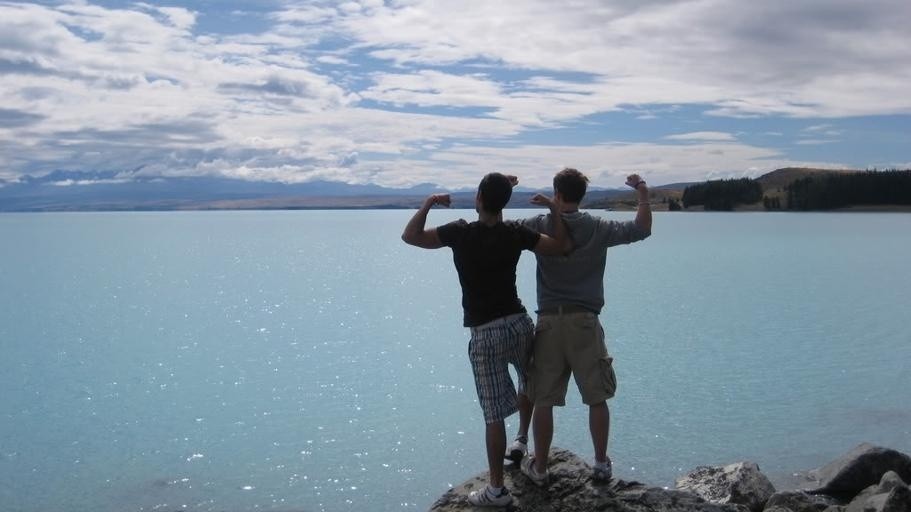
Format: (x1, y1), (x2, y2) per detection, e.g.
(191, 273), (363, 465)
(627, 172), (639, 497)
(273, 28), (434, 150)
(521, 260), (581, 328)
(499, 167), (654, 491)
(401, 170), (579, 507)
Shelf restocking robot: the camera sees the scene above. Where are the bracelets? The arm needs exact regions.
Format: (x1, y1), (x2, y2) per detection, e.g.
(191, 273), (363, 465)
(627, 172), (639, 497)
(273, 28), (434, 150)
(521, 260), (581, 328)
(634, 180), (648, 190)
(552, 214), (561, 219)
(433, 193), (442, 206)
(639, 201), (651, 206)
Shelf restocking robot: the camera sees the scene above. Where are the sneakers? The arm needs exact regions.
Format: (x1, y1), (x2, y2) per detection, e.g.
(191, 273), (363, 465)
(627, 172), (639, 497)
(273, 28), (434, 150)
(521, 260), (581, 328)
(468, 483), (515, 509)
(591, 454), (614, 482)
(503, 435), (529, 463)
(517, 454), (552, 489)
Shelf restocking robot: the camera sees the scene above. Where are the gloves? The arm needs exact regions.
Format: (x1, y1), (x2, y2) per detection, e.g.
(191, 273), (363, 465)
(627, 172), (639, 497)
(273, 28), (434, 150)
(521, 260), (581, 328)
(625, 173), (646, 190)
